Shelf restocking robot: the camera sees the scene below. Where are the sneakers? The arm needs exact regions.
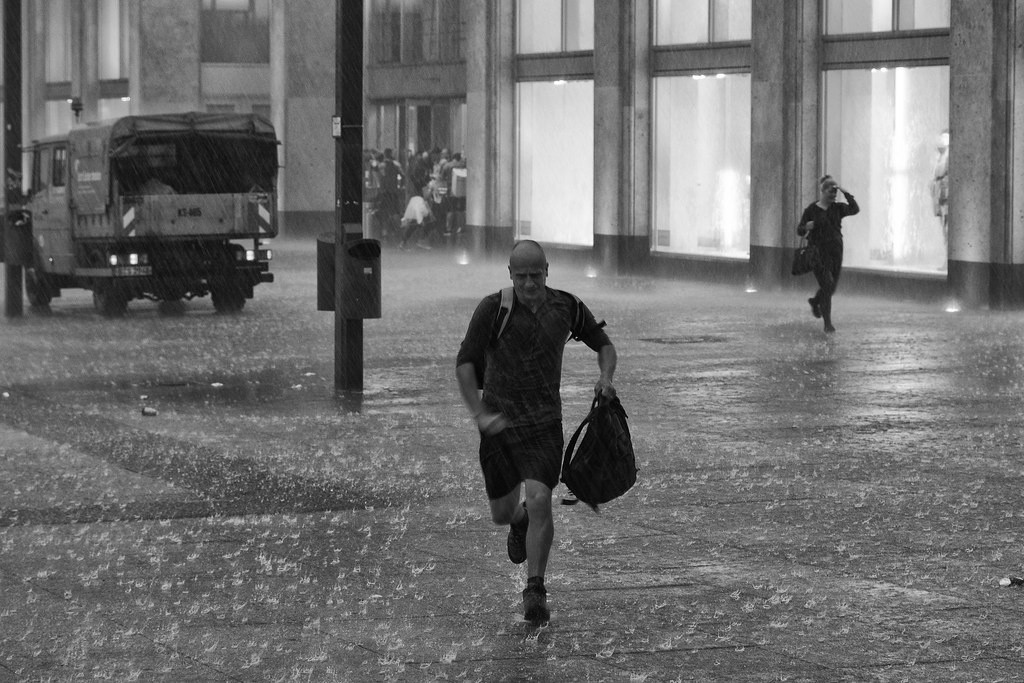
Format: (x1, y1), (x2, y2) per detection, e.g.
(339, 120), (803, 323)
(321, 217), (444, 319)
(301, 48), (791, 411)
(508, 501), (529, 565)
(522, 587), (548, 623)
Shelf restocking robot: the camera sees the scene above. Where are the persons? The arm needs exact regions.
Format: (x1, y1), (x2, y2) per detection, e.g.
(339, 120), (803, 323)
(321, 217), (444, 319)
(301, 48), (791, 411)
(456, 240), (619, 618)
(797, 175), (861, 335)
(362, 148), (467, 252)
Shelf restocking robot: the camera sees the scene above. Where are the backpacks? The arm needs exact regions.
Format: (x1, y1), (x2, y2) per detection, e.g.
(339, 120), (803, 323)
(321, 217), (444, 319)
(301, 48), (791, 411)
(560, 386), (635, 513)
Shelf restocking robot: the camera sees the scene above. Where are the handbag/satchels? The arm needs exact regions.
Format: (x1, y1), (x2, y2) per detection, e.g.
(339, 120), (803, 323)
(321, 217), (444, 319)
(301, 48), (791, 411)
(792, 227), (813, 277)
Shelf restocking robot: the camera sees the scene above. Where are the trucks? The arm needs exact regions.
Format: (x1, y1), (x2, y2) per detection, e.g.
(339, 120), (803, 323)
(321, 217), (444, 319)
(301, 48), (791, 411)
(17, 111), (280, 316)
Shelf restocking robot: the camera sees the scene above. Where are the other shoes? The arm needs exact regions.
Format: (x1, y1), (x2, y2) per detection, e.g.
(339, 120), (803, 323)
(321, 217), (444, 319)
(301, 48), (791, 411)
(418, 241), (431, 250)
(399, 241), (408, 250)
(809, 298), (822, 319)
(825, 325), (835, 334)
